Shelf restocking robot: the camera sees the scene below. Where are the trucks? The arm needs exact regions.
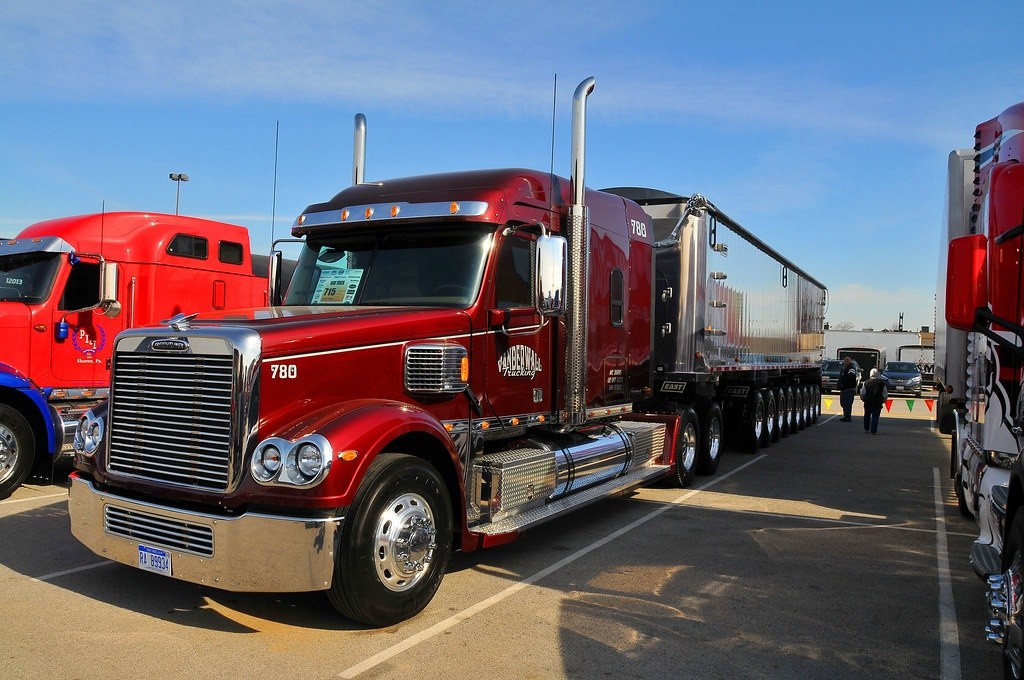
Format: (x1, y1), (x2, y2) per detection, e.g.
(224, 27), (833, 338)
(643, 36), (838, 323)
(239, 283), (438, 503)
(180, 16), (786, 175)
(0, 211), (341, 502)
(936, 95), (1024, 680)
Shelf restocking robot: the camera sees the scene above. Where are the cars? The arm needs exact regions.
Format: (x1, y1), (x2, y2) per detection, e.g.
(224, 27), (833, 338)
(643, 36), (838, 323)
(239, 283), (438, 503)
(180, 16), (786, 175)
(879, 360), (923, 398)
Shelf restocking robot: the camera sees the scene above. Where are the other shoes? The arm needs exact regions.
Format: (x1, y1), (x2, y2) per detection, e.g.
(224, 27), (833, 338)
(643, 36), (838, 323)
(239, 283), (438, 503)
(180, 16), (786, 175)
(840, 415), (851, 422)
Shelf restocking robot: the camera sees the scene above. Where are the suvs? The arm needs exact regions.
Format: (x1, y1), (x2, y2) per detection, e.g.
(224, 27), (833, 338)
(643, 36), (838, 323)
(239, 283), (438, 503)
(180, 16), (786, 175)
(822, 358), (865, 396)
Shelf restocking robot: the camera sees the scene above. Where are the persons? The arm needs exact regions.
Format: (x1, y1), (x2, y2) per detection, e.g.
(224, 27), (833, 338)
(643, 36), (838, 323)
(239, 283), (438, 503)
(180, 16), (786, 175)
(860, 367), (888, 434)
(837, 357), (857, 422)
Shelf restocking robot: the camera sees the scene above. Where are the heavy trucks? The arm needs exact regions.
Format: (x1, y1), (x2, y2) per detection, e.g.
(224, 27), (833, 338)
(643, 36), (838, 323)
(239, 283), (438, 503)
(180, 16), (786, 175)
(66, 59), (830, 627)
